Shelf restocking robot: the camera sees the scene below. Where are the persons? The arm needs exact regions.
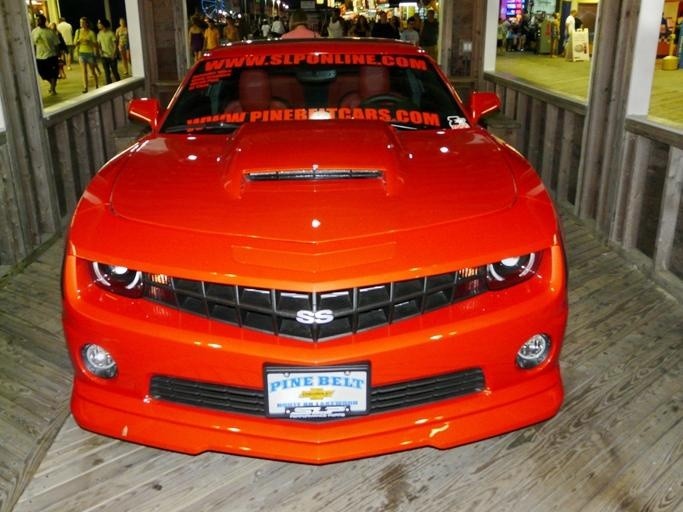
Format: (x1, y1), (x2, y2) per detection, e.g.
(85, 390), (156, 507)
(31, 15), (131, 95)
(187, 8), (439, 64)
(497, 8), (583, 62)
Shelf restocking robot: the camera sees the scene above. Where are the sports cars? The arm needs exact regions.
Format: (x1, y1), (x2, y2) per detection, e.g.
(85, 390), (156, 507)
(62, 38), (570, 459)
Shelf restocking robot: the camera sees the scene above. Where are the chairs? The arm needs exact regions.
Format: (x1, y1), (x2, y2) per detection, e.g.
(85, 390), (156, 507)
(218, 70), (288, 115)
(327, 76), (358, 106)
(267, 74), (304, 106)
(338, 64), (409, 109)
